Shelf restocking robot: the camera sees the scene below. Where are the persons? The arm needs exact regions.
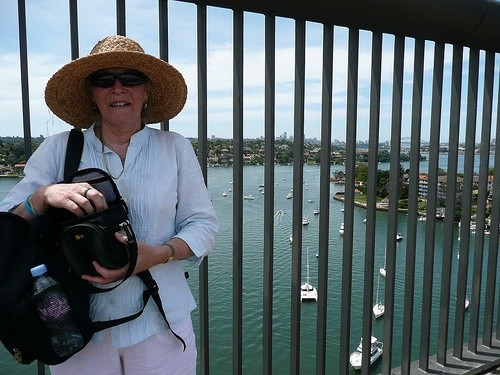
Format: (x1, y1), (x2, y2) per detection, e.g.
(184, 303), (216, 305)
(0, 36), (220, 375)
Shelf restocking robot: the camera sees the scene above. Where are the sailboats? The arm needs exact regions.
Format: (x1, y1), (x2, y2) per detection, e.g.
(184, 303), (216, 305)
(300, 245), (318, 302)
(372, 274), (385, 319)
(379, 245), (387, 278)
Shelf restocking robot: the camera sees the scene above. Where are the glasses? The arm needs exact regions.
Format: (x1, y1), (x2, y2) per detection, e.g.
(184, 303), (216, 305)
(86, 71), (149, 87)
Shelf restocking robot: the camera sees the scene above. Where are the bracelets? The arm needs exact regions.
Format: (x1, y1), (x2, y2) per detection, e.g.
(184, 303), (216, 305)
(24, 192), (38, 217)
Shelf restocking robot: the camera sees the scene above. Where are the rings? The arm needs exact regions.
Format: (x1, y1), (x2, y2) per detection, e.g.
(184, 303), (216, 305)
(84, 187), (90, 196)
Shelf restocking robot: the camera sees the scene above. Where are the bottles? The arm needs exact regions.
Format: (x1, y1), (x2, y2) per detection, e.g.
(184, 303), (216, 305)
(30, 263), (84, 359)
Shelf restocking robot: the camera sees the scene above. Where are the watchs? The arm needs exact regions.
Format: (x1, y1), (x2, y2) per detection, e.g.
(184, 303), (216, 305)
(162, 241), (175, 264)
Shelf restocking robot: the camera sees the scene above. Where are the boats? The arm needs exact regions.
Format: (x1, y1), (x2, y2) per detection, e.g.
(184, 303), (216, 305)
(313, 206), (320, 215)
(464, 295), (470, 310)
(349, 335), (383, 371)
(456, 218), (490, 260)
(417, 214), (426, 222)
(222, 165), (316, 203)
(338, 222), (345, 237)
(302, 217), (310, 226)
(396, 232), (403, 242)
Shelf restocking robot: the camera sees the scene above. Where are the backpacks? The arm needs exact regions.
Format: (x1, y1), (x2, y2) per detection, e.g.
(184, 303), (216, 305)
(0, 141), (157, 367)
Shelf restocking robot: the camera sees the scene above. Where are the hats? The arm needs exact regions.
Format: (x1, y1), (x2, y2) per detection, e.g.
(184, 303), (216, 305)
(43, 34), (187, 129)
